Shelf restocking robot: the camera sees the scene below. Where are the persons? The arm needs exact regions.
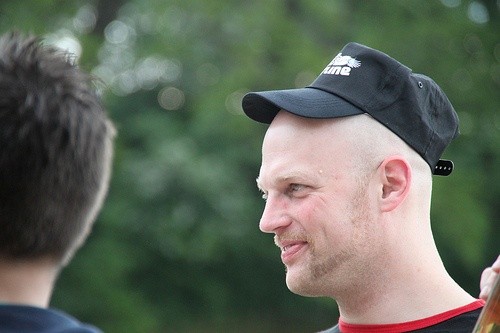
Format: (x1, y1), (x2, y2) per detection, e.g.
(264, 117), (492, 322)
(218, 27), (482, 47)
(1, 31), (115, 332)
(242, 42), (500, 333)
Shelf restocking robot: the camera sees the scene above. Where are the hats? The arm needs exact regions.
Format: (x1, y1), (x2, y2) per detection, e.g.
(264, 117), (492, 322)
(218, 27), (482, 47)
(242, 41), (459, 176)
